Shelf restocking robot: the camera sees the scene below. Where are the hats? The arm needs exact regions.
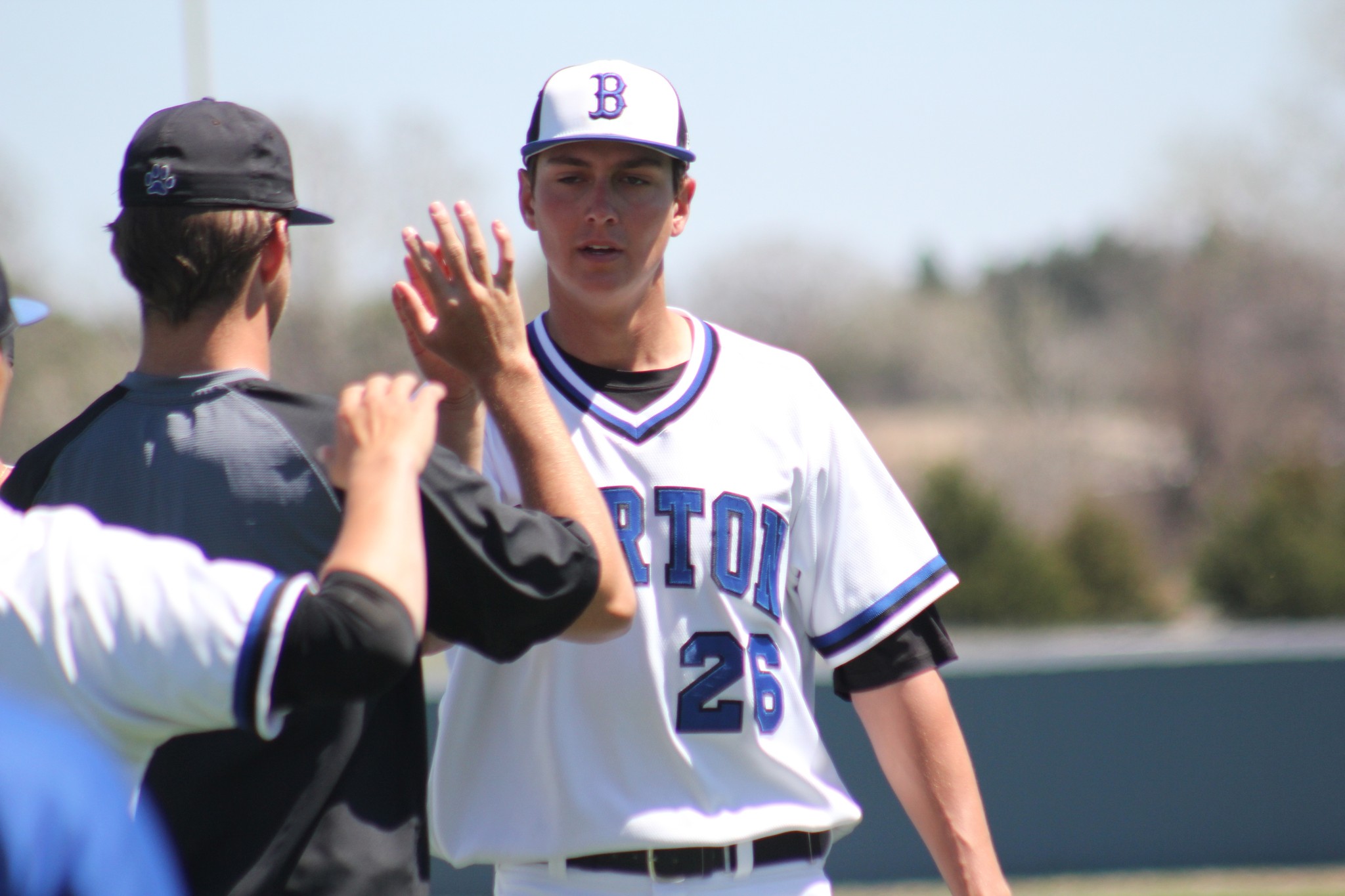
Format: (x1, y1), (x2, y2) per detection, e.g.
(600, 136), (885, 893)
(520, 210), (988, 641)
(521, 58), (695, 172)
(118, 96), (335, 226)
(0, 267), (51, 337)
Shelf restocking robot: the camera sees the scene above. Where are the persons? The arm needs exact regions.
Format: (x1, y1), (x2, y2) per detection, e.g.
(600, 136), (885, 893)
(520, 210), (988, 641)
(0, 260), (446, 896)
(392, 59), (1012, 896)
(0, 97), (637, 896)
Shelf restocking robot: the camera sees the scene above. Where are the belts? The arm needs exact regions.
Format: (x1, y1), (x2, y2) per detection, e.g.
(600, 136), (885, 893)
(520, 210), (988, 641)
(539, 828), (834, 885)
